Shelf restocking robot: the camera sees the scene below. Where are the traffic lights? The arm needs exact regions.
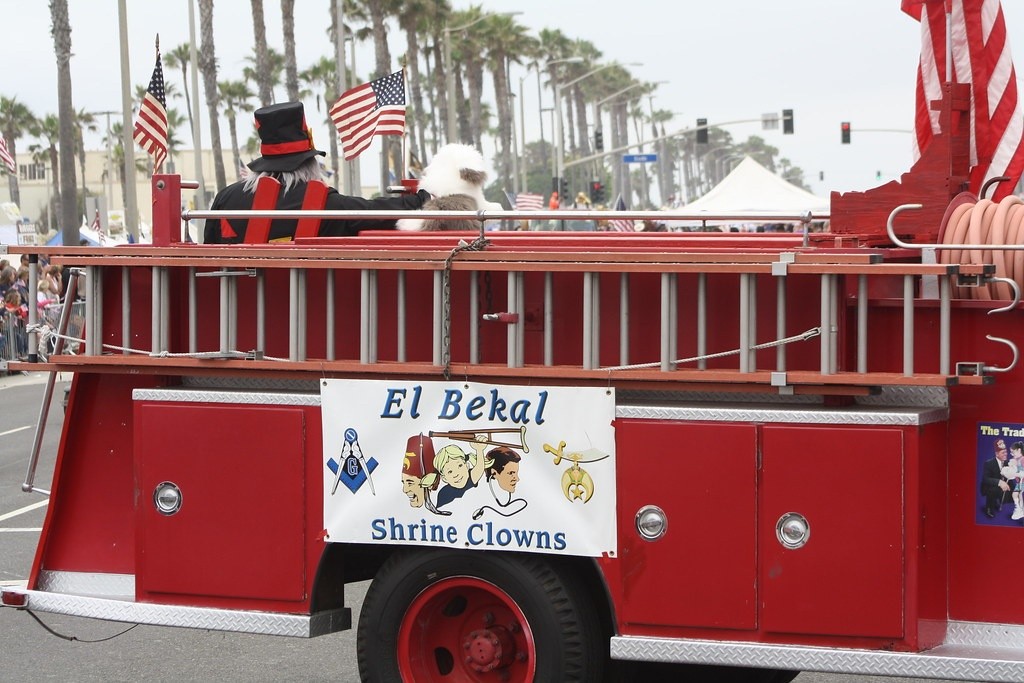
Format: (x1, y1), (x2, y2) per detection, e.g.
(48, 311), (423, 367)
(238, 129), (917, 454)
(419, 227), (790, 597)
(819, 171), (824, 181)
(550, 175), (568, 200)
(782, 109), (794, 135)
(594, 132), (603, 150)
(842, 123), (851, 143)
(877, 171), (881, 180)
(695, 117), (709, 147)
(590, 180), (603, 202)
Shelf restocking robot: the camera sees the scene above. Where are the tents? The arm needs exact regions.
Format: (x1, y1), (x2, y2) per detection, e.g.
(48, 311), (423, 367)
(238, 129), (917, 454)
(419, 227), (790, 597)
(45, 225), (116, 247)
(669, 155), (831, 226)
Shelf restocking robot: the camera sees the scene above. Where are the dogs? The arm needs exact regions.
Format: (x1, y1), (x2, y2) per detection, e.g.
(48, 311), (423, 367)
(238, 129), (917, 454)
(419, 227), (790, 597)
(393, 141), (489, 231)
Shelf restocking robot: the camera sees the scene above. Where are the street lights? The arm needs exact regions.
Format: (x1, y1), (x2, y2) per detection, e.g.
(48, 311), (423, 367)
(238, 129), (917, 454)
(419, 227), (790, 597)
(555, 60), (642, 199)
(515, 53), (585, 195)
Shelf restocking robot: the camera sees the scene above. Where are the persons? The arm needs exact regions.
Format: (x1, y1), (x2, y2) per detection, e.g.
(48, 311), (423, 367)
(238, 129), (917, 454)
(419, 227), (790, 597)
(982, 438), (1024, 519)
(0, 254), (70, 360)
(203, 103), (430, 245)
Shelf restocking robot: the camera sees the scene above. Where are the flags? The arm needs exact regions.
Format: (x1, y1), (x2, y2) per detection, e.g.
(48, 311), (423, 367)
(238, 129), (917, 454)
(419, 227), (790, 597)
(91, 213), (106, 242)
(133, 48), (168, 175)
(901, 0), (1024, 204)
(329, 70), (405, 161)
(515, 193), (544, 210)
(609, 195), (635, 232)
(0, 137), (15, 172)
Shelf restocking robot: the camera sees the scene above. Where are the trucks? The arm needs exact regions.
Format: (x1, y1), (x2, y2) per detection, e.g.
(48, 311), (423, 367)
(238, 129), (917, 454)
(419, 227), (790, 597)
(0, 82), (1023, 683)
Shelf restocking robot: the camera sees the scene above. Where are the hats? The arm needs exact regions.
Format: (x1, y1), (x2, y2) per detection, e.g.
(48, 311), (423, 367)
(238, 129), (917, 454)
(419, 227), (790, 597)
(247, 103), (326, 172)
